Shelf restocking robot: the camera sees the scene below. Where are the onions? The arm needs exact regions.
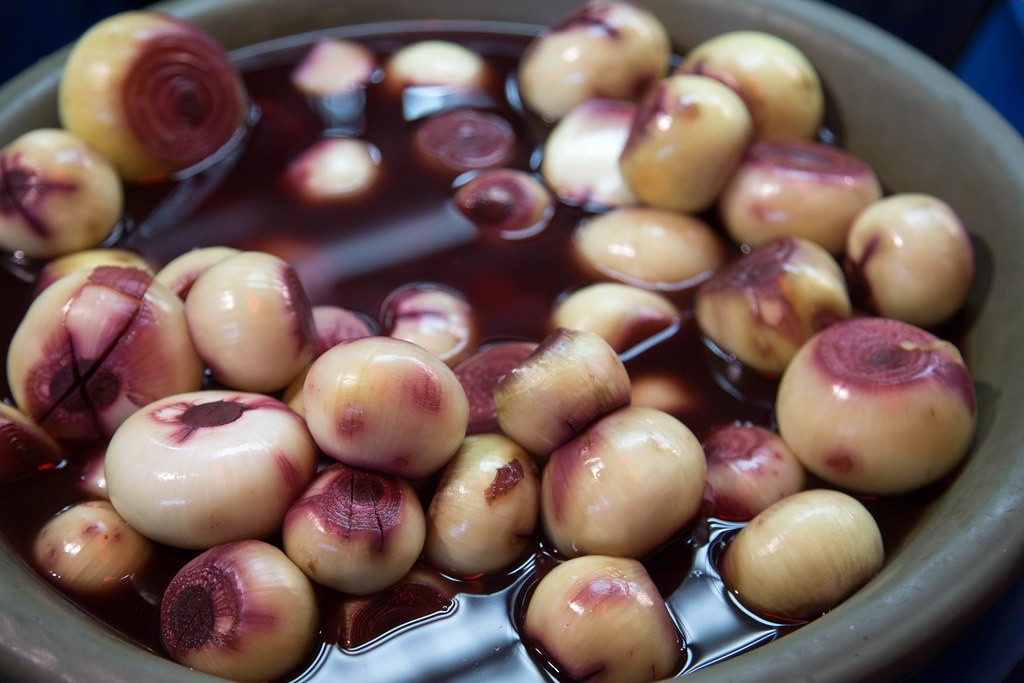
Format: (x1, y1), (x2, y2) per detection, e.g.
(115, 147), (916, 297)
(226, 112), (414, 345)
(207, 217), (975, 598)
(0, 6), (977, 683)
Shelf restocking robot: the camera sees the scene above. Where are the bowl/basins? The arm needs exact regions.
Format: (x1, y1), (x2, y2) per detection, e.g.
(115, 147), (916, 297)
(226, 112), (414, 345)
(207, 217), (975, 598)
(0, 0), (1024, 683)
